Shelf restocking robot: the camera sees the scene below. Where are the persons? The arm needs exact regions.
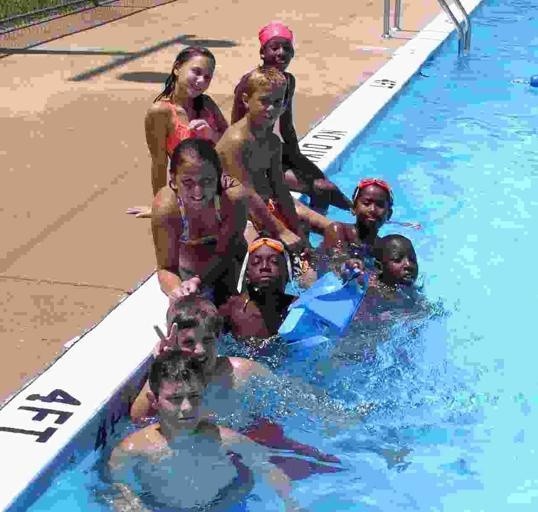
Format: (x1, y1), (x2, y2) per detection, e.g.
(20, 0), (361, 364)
(374, 234), (419, 286)
(320, 177), (393, 273)
(130, 294), (275, 426)
(231, 25), (354, 219)
(215, 64), (317, 267)
(214, 231), (298, 340)
(127, 46), (332, 245)
(149, 135), (287, 325)
(110, 350), (293, 512)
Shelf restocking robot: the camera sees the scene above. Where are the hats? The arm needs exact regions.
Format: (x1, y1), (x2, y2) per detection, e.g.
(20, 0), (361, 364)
(258, 23), (295, 45)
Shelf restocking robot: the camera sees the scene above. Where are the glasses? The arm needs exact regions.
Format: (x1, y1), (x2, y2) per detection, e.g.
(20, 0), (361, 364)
(248, 237), (284, 252)
(354, 177), (390, 190)
